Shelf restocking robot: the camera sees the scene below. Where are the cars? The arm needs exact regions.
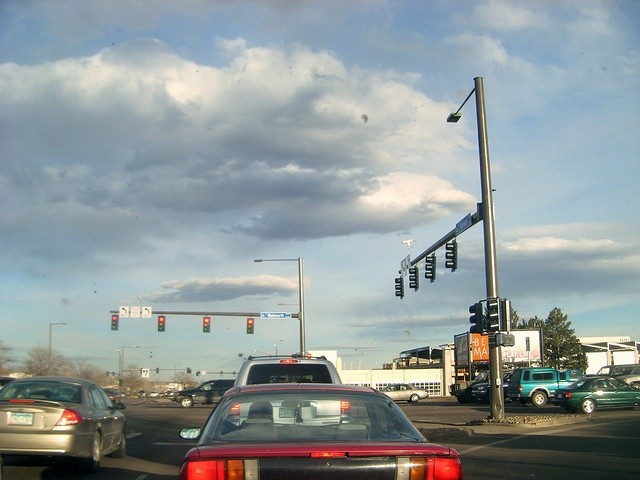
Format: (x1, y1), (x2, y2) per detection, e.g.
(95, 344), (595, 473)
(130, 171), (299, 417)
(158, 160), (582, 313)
(551, 374), (640, 414)
(382, 384), (428, 403)
(179, 384), (464, 480)
(0, 376), (126, 472)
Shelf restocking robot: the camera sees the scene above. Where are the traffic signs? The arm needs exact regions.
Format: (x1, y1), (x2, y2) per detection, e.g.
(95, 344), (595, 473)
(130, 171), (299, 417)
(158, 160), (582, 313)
(455, 213), (471, 236)
(261, 312), (292, 320)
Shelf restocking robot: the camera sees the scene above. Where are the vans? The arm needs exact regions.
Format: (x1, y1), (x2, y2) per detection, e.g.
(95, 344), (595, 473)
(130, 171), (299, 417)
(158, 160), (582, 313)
(234, 355), (342, 386)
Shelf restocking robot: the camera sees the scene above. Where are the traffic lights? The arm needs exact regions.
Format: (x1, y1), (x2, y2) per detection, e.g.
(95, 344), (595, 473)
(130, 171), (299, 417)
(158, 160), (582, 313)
(111, 315), (119, 330)
(469, 303), (483, 333)
(445, 242), (457, 272)
(396, 278), (404, 299)
(409, 268), (419, 291)
(247, 318), (254, 334)
(203, 317), (211, 333)
(158, 316), (165, 332)
(425, 256), (436, 282)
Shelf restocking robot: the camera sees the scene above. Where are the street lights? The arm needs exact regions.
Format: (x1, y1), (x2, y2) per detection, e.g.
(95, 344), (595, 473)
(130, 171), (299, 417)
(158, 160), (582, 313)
(117, 350), (120, 378)
(254, 257), (305, 359)
(447, 77), (505, 421)
(48, 323), (67, 357)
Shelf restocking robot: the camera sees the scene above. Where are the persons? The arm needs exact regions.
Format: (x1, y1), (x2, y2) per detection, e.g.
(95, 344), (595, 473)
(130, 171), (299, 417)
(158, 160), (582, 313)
(222, 402), (273, 433)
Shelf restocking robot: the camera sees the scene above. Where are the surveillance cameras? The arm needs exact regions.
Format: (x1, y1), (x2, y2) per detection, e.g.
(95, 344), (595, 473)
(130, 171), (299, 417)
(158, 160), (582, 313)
(401, 239), (414, 244)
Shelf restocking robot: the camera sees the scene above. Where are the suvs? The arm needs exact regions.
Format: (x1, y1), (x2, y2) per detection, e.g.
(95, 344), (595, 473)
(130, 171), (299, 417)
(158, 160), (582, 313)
(616, 367), (640, 390)
(507, 368), (584, 407)
(472, 372), (520, 402)
(174, 380), (236, 408)
